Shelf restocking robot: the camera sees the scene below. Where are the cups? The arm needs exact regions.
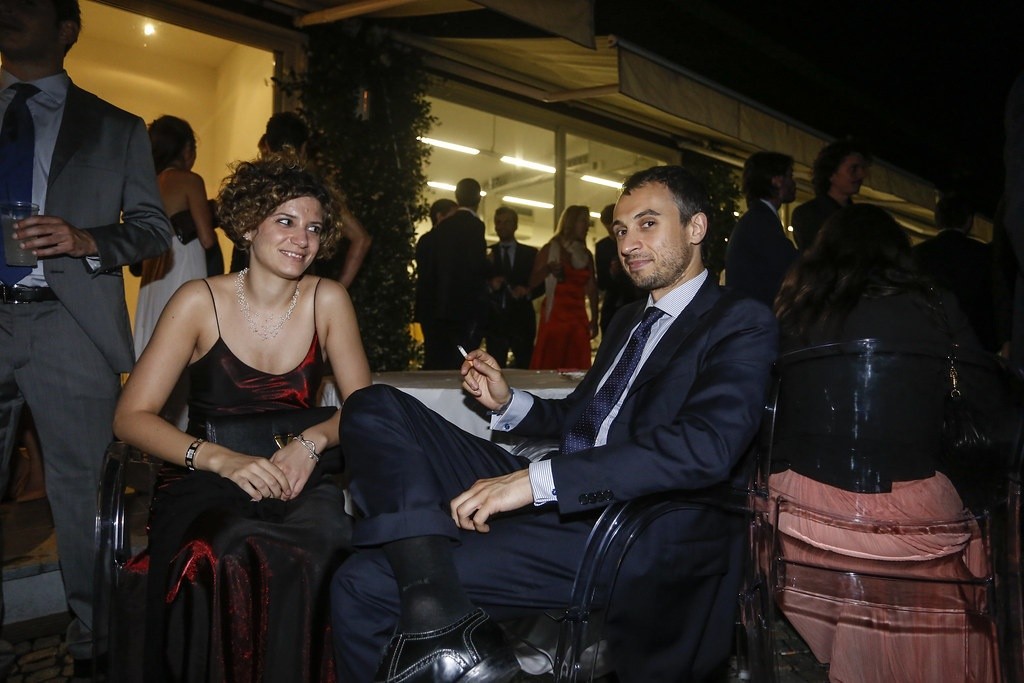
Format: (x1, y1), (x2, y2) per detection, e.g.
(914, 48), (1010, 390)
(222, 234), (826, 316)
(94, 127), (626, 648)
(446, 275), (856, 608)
(1, 201), (40, 265)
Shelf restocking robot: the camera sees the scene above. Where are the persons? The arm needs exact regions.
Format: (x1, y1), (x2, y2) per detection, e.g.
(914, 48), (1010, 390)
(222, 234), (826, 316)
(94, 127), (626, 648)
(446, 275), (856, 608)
(110, 142), (373, 683)
(724, 139), (874, 305)
(527, 205), (599, 370)
(484, 204), (546, 370)
(132, 113), (219, 433)
(228, 111), (375, 289)
(412, 177), (503, 371)
(911, 191), (1003, 356)
(596, 205), (650, 339)
(0, 0), (173, 683)
(330, 164), (780, 683)
(757, 202), (1006, 485)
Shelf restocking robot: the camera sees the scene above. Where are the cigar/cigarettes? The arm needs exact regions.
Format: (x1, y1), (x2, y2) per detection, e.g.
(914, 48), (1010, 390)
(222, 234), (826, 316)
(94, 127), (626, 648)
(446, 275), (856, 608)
(457, 344), (474, 366)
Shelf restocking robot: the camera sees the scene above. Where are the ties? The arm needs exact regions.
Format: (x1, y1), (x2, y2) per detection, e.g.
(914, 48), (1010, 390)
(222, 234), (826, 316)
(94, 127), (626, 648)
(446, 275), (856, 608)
(561, 306), (665, 456)
(0, 81), (40, 288)
(503, 242), (512, 282)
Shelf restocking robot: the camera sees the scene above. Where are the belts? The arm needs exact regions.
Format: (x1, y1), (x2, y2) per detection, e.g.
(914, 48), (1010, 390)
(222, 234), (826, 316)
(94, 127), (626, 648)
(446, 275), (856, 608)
(0, 283), (58, 304)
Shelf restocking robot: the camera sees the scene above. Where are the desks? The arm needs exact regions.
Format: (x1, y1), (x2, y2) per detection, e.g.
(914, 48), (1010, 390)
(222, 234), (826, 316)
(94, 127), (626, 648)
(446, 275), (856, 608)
(321, 369), (587, 441)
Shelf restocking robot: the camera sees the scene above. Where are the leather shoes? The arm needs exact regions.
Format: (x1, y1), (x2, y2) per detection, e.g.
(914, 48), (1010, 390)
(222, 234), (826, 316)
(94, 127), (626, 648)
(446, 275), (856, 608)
(374, 607), (521, 683)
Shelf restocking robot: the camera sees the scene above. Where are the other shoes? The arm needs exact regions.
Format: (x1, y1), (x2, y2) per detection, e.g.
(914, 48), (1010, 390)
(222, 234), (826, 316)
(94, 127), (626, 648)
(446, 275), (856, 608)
(73, 650), (110, 683)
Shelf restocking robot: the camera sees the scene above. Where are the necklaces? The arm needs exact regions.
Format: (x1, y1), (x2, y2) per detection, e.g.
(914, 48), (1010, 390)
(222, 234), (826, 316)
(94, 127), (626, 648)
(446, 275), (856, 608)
(234, 265), (300, 341)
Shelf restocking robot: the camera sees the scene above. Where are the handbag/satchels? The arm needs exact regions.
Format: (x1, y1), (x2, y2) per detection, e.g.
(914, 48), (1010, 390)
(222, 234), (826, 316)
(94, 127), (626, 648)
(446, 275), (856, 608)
(206, 411), (344, 477)
(944, 351), (994, 466)
(170, 198), (221, 246)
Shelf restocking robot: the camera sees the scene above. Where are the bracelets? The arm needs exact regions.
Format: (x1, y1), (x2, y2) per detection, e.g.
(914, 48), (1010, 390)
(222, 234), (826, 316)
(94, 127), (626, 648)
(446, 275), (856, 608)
(185, 438), (208, 472)
(293, 434), (321, 462)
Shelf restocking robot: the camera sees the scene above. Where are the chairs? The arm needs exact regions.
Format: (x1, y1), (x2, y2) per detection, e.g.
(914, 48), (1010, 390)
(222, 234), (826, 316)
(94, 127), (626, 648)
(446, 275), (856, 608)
(504, 339), (1024, 683)
(93, 413), (178, 683)
